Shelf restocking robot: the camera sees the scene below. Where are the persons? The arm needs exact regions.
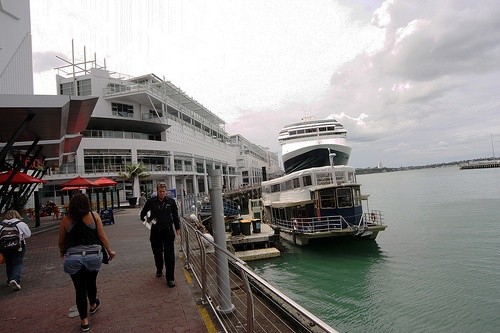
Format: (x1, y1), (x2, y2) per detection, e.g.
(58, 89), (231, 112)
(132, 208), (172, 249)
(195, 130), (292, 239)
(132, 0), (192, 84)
(39, 200), (55, 215)
(0, 210), (31, 289)
(167, 190), (181, 220)
(151, 188), (157, 197)
(59, 193), (115, 331)
(139, 192), (147, 209)
(141, 183), (180, 288)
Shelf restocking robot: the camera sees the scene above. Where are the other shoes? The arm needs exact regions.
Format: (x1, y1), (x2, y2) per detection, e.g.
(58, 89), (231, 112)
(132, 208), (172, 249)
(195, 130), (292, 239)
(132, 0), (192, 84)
(90, 298), (101, 313)
(10, 279), (21, 290)
(80, 322), (90, 331)
(167, 280), (175, 287)
(156, 270), (162, 277)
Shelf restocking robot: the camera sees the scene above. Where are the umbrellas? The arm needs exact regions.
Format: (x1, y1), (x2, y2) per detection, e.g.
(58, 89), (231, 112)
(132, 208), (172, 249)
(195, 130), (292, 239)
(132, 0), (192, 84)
(0, 170), (46, 212)
(61, 176), (117, 214)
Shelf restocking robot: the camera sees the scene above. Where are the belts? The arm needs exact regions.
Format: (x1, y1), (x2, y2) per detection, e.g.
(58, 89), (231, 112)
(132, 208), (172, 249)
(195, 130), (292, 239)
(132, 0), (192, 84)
(70, 250), (99, 254)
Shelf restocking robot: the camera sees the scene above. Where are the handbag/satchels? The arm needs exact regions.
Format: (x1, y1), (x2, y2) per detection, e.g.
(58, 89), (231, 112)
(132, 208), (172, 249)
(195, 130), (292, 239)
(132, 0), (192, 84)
(100, 245), (109, 264)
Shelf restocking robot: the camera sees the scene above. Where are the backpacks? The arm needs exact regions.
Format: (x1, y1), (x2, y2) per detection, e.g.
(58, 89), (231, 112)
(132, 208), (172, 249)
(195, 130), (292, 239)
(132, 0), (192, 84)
(0, 220), (23, 252)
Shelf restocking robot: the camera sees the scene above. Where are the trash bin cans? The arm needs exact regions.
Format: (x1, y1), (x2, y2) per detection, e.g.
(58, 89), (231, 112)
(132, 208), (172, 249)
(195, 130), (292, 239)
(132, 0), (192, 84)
(229, 219), (262, 236)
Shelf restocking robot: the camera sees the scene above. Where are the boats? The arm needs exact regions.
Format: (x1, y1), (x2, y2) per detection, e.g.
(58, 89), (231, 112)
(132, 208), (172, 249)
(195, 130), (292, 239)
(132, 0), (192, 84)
(276, 119), (352, 175)
(260, 166), (387, 246)
